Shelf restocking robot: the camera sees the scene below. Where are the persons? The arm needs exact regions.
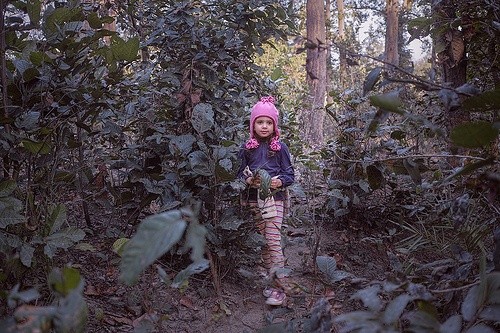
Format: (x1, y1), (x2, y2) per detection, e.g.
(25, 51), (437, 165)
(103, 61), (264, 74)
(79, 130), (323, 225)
(235, 97), (293, 304)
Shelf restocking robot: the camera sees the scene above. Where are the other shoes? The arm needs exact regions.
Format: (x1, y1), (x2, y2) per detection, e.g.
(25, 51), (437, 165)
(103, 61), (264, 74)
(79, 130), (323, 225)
(265, 289), (286, 305)
(263, 285), (274, 297)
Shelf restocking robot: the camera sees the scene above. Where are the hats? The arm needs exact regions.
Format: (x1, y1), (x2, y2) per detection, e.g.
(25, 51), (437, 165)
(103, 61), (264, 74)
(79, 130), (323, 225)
(246, 96), (281, 151)
